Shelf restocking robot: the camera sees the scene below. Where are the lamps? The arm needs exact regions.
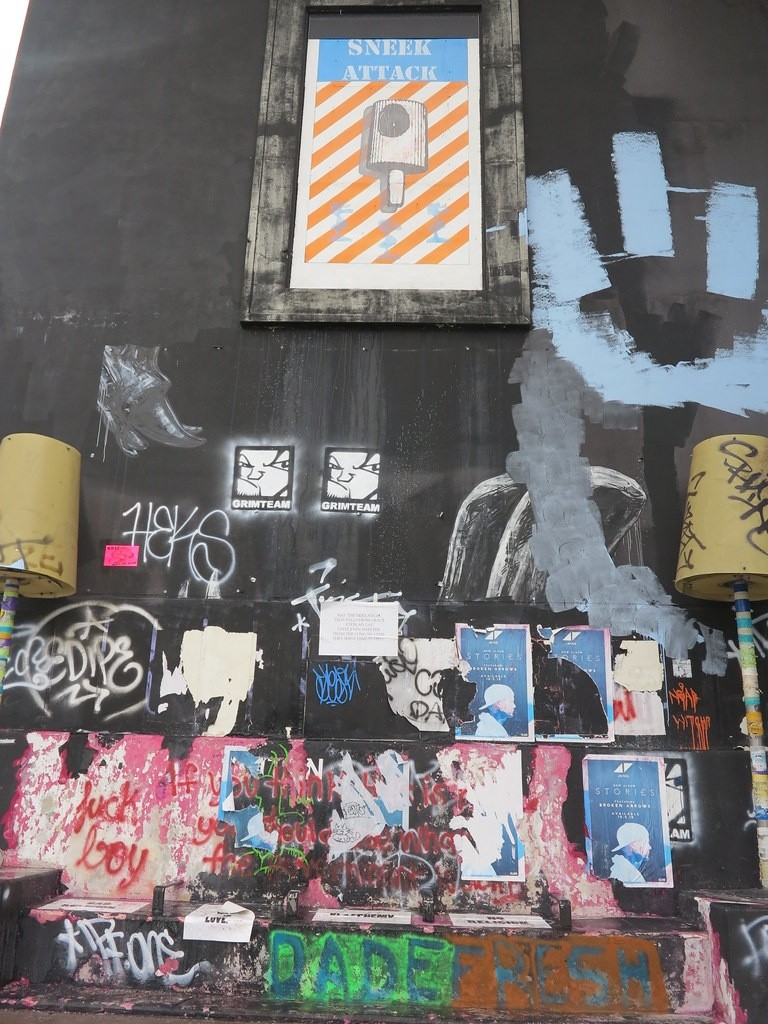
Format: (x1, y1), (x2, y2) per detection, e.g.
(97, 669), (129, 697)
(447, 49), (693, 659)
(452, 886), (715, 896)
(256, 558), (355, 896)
(0, 431), (81, 714)
(673, 432), (768, 891)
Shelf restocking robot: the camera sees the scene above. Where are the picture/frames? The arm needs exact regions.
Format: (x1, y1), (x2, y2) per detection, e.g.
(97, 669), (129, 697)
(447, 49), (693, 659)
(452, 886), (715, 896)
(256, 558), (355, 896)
(240, 0), (531, 326)
